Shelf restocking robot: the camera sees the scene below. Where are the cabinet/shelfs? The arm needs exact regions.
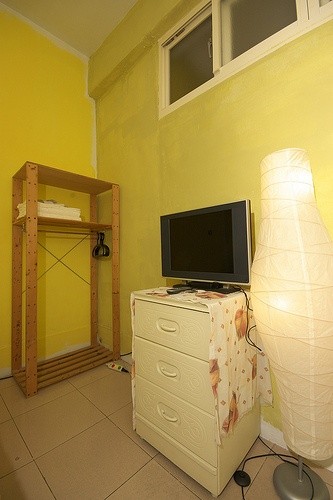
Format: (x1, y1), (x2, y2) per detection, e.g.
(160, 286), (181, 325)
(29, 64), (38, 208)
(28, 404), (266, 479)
(129, 287), (274, 497)
(11, 160), (121, 398)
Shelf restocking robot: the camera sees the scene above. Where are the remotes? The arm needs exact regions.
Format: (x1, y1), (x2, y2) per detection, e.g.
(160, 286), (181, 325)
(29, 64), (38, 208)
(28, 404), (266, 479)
(166, 287), (191, 294)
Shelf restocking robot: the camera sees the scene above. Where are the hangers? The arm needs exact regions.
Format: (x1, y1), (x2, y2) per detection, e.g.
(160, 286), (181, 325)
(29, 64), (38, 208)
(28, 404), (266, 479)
(92, 232), (110, 258)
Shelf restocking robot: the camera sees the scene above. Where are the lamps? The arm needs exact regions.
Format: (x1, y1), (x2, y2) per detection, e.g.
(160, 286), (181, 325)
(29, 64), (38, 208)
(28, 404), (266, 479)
(250, 148), (333, 500)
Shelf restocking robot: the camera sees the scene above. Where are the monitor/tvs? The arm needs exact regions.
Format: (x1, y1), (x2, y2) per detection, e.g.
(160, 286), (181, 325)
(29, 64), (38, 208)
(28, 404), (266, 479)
(160, 200), (252, 294)
